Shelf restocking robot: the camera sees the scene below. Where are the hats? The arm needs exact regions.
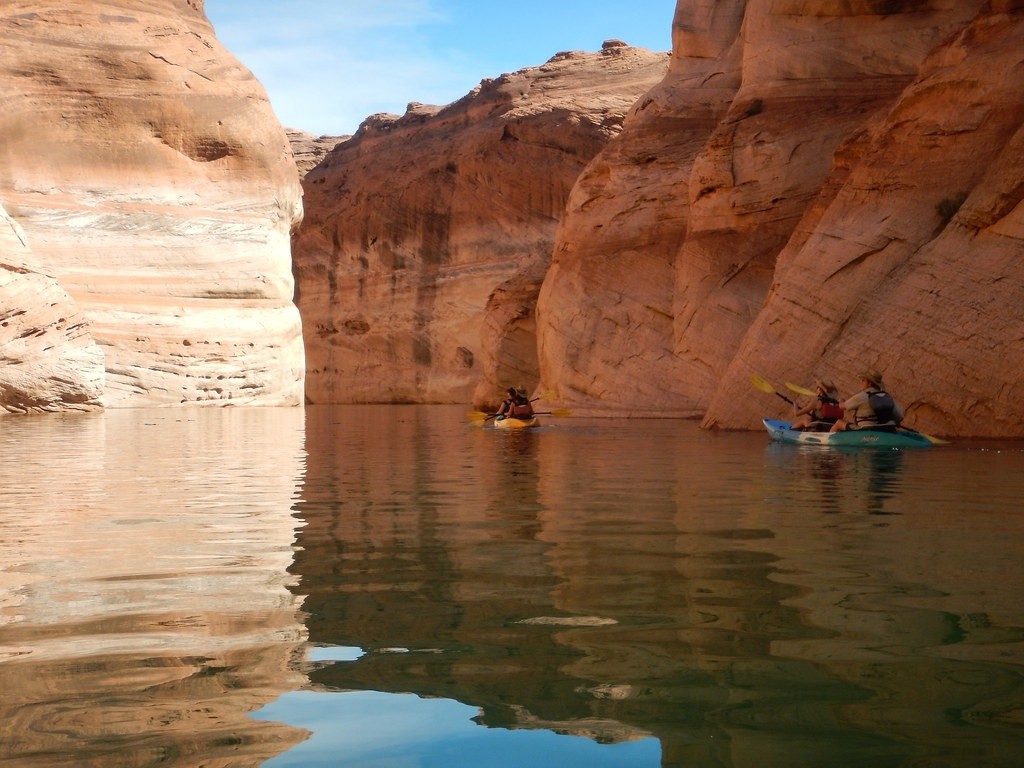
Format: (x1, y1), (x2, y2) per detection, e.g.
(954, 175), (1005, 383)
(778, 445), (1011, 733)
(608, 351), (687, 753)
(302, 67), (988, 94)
(506, 387), (516, 397)
(516, 385), (528, 398)
(816, 380), (838, 400)
(857, 369), (883, 387)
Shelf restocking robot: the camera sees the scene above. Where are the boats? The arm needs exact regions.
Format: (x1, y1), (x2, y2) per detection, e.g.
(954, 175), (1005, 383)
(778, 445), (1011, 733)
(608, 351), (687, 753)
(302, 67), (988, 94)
(761, 417), (934, 449)
(494, 415), (541, 430)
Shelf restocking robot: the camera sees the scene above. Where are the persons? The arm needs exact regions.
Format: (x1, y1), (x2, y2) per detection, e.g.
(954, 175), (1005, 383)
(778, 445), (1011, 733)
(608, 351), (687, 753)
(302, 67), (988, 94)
(828, 368), (901, 432)
(496, 387), (516, 419)
(790, 377), (839, 435)
(505, 385), (534, 421)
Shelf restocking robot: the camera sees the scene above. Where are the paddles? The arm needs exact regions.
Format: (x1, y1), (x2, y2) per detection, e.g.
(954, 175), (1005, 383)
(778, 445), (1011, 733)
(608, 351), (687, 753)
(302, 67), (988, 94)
(785, 381), (946, 446)
(751, 374), (813, 417)
(465, 407), (574, 421)
(472, 390), (556, 427)
